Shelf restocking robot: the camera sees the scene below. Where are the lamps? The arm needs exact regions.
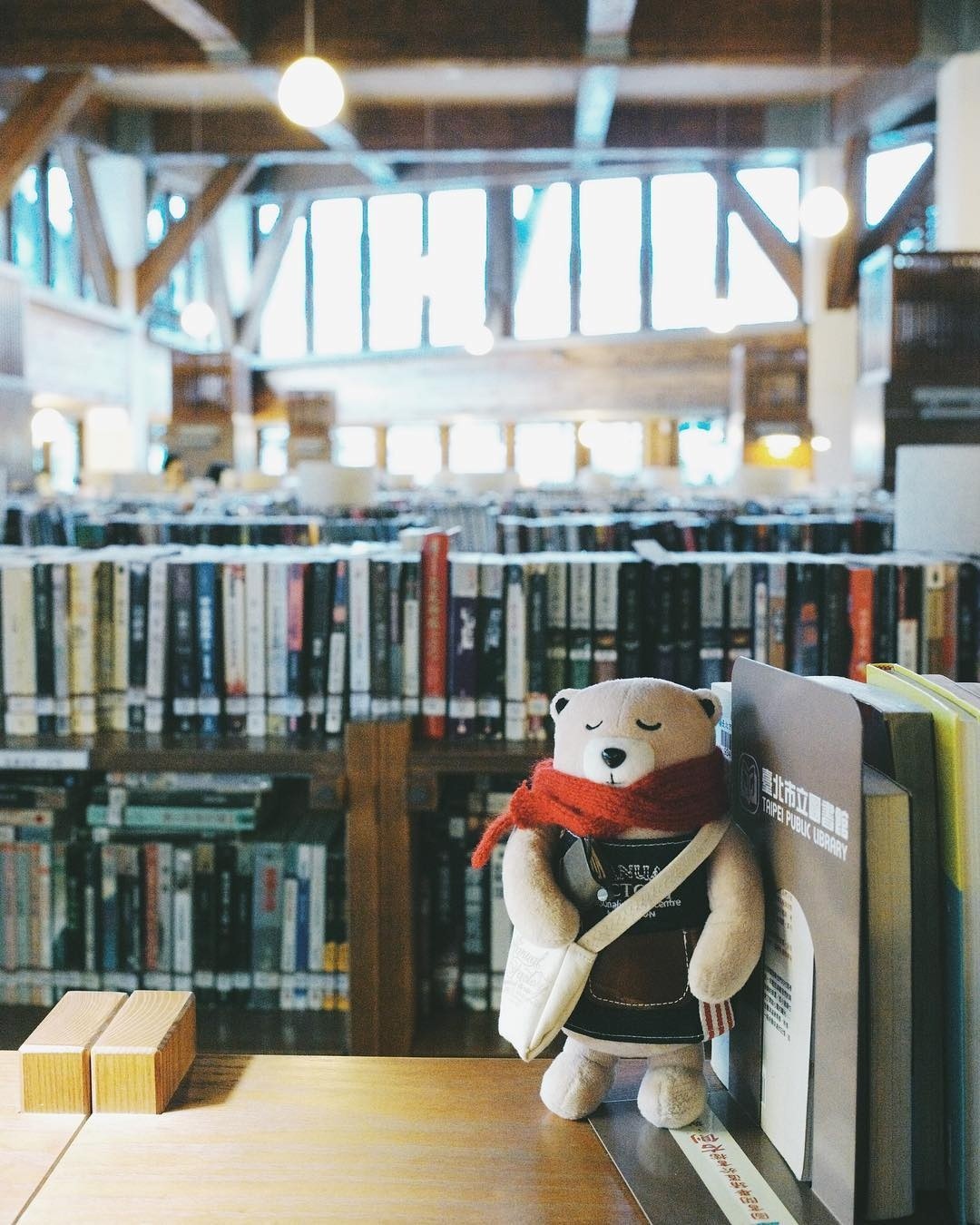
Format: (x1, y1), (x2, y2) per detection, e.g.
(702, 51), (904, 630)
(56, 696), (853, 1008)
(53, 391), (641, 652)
(276, 0), (345, 130)
(797, 0), (850, 238)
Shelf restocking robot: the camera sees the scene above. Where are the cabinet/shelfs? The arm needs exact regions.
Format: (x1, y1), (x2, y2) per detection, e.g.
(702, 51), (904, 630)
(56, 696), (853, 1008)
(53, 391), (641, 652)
(0, 241), (980, 1225)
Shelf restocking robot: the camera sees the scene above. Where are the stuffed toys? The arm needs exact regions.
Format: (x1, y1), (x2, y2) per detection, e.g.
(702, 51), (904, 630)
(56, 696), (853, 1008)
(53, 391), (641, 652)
(473, 677), (767, 1130)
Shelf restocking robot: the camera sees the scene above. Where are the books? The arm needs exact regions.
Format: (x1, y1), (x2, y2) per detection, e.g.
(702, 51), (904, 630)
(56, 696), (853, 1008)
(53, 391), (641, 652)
(1, 492), (980, 1225)
